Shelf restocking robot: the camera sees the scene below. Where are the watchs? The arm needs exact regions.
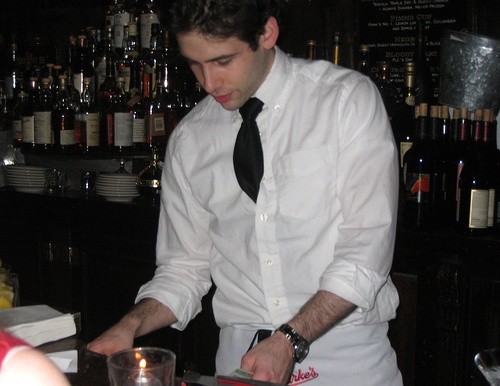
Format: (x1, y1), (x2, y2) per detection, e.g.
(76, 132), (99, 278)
(273, 324), (310, 364)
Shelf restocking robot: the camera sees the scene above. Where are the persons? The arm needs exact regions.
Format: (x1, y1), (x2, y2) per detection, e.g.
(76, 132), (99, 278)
(87, 0), (402, 386)
(0, 330), (70, 386)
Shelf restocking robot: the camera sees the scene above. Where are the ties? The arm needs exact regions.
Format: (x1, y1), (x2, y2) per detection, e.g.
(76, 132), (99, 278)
(232, 97), (264, 204)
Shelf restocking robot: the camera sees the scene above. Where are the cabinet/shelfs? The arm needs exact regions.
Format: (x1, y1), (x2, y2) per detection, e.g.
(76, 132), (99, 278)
(390, 224), (500, 386)
(0, 185), (166, 340)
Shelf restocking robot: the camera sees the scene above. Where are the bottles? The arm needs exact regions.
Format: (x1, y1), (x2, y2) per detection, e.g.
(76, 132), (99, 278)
(304, 31), (500, 232)
(0, 0), (174, 157)
(140, 153), (162, 198)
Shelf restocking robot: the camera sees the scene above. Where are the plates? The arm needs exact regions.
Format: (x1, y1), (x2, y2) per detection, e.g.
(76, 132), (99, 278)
(4, 167), (47, 193)
(96, 173), (140, 203)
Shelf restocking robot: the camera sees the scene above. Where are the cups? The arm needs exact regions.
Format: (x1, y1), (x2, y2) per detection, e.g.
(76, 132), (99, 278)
(107, 347), (176, 386)
(474, 348), (500, 386)
